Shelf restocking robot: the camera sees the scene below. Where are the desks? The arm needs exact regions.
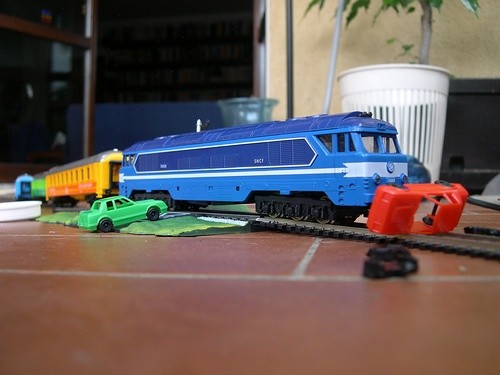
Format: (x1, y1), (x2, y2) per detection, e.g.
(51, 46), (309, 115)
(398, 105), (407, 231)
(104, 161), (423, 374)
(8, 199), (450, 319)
(64, 102), (222, 165)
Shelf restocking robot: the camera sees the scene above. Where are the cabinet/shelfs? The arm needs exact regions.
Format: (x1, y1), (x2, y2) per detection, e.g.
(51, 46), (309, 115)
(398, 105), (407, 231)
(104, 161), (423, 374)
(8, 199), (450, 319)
(97, 34), (253, 104)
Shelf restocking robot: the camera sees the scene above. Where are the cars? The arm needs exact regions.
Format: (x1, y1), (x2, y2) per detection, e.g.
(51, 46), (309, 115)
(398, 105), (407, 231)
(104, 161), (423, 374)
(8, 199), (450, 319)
(77, 194), (171, 233)
(365, 181), (469, 236)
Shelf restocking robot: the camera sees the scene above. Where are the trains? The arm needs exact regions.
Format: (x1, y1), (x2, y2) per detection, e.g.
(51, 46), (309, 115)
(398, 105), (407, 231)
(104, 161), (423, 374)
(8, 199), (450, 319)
(13, 110), (412, 225)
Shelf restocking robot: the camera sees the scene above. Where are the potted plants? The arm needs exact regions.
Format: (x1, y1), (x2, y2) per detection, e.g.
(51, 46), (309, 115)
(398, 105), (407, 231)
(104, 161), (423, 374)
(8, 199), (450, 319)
(302, 0), (481, 185)
